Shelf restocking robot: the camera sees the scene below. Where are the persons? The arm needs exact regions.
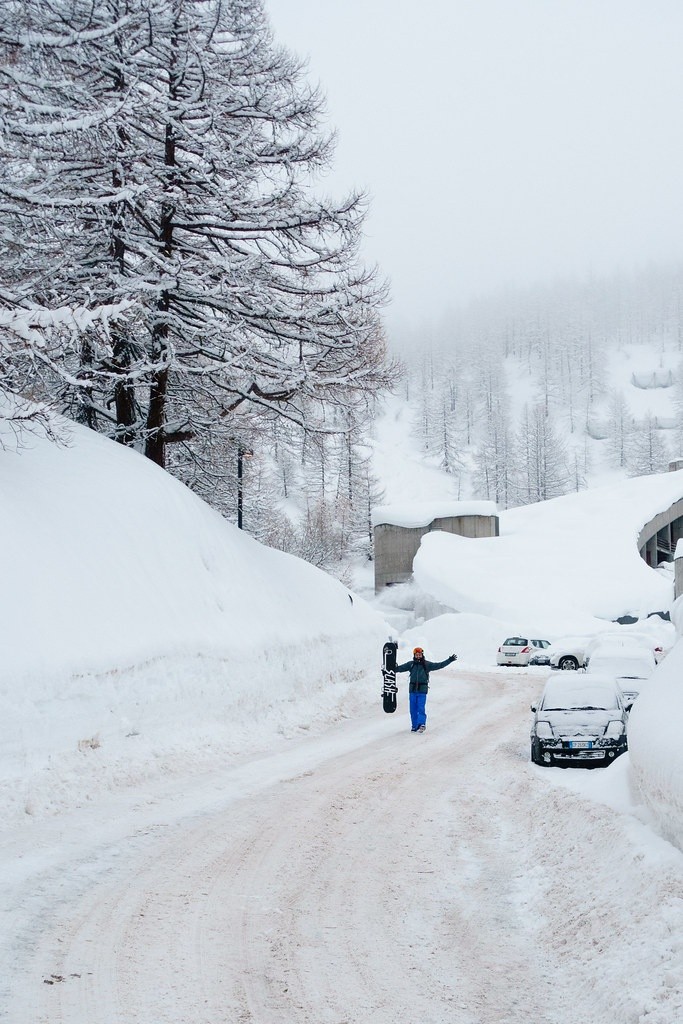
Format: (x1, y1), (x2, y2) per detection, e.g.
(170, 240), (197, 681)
(381, 647), (458, 733)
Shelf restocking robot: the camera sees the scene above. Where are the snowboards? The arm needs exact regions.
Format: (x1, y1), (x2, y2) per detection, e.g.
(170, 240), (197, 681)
(381, 642), (399, 713)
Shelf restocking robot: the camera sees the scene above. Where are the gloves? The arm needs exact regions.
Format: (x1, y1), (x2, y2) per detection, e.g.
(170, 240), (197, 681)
(449, 654), (457, 663)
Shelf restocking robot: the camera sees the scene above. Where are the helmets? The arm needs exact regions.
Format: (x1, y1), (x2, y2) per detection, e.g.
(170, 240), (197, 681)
(413, 648), (422, 654)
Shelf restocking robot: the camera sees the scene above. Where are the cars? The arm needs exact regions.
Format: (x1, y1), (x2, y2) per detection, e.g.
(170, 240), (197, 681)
(530, 674), (633, 769)
(496, 635), (554, 667)
(529, 635), (668, 709)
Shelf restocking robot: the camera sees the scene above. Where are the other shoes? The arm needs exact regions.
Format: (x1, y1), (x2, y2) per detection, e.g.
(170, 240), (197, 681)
(411, 730), (417, 732)
(420, 725), (425, 733)
